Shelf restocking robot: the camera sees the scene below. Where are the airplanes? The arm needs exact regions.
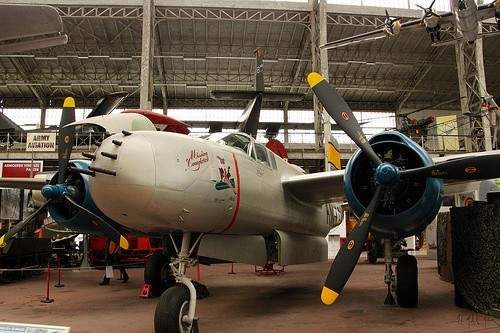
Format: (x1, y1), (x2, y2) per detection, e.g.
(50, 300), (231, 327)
(346, 94), (467, 148)
(0, 77), (129, 152)
(0, 70), (500, 333)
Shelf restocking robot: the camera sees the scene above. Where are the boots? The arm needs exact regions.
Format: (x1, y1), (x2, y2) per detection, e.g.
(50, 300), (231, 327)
(122, 274), (129, 283)
(99, 277), (110, 285)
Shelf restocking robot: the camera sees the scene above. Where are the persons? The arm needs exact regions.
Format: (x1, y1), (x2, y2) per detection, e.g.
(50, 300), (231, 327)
(99, 267), (130, 285)
